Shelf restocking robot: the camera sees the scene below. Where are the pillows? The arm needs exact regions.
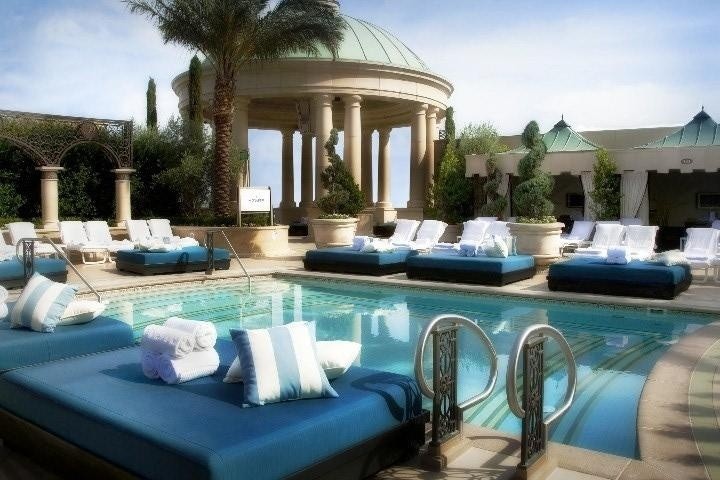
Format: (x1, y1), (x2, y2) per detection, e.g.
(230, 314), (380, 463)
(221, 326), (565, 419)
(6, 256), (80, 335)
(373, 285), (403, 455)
(8, 271), (76, 334)
(222, 338), (362, 382)
(657, 250), (690, 267)
(361, 241), (387, 253)
(56, 299), (107, 326)
(485, 243), (509, 258)
(229, 319), (342, 409)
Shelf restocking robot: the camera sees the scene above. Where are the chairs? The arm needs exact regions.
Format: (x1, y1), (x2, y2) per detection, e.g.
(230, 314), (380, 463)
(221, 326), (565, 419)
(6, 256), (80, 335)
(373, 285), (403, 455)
(387, 219), (449, 248)
(682, 227), (720, 284)
(454, 218), (513, 249)
(560, 220), (660, 260)
(0, 219), (181, 265)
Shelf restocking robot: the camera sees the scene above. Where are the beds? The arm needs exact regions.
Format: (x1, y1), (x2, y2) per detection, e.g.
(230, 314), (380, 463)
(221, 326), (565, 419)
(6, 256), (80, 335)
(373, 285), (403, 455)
(548, 255), (693, 301)
(0, 336), (431, 479)
(0, 254), (67, 290)
(303, 245), (418, 277)
(405, 249), (536, 286)
(1, 301), (133, 378)
(117, 245), (232, 276)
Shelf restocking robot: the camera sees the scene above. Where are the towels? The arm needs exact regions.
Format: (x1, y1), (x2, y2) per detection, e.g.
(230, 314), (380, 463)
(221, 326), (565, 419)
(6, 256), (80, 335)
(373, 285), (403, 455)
(141, 316), (222, 384)
(181, 237), (200, 248)
(351, 235), (371, 250)
(1, 284), (10, 320)
